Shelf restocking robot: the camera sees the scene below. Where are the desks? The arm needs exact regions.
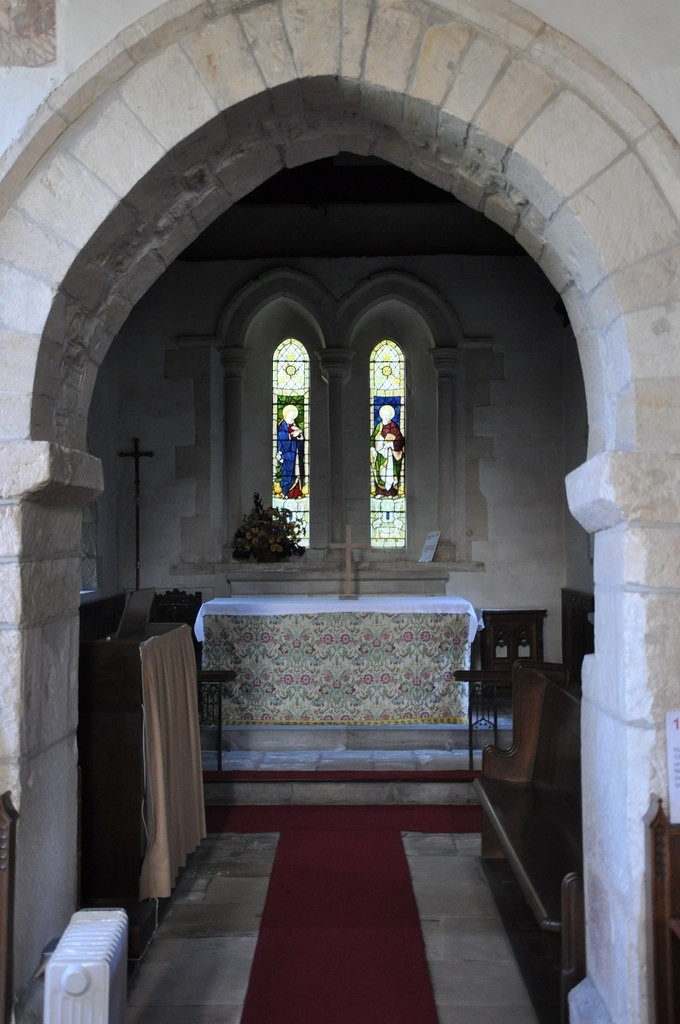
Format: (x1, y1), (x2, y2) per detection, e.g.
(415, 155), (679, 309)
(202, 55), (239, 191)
(195, 597), (476, 727)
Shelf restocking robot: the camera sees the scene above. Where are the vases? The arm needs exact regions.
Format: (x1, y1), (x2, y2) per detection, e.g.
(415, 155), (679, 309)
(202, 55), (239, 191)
(254, 543), (279, 563)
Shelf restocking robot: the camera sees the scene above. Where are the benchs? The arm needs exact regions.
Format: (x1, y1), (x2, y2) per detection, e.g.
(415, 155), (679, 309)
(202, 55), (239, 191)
(473, 660), (590, 1024)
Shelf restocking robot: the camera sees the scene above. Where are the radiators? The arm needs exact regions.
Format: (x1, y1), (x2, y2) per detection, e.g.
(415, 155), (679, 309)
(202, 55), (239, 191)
(43, 907), (128, 1024)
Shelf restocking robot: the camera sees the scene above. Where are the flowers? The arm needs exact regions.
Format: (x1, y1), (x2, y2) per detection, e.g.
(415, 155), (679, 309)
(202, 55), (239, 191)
(232, 492), (308, 561)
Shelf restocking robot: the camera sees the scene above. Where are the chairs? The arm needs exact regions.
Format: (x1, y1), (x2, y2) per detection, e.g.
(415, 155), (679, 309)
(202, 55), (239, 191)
(482, 607), (548, 744)
